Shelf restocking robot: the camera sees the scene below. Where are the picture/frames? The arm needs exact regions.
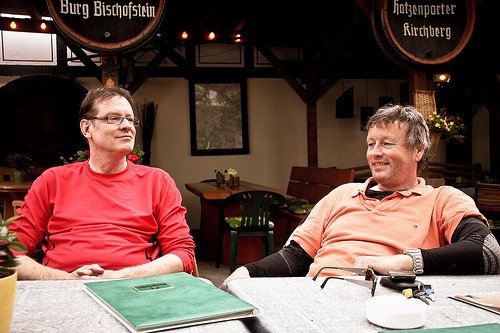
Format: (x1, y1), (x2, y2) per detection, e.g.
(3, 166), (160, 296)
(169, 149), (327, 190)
(188, 78), (250, 156)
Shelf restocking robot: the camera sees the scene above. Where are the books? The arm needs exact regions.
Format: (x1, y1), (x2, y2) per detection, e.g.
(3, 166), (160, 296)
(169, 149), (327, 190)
(84, 271), (259, 333)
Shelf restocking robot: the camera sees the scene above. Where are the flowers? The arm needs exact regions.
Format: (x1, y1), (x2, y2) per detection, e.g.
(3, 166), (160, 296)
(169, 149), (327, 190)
(424, 106), (466, 144)
(214, 167), (239, 177)
(0, 214), (28, 268)
(6, 152), (36, 174)
(60, 144), (145, 166)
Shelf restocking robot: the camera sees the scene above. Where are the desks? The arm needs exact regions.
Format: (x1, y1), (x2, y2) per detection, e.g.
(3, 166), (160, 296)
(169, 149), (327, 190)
(184, 178), (282, 265)
(9, 277), (251, 333)
(220, 274), (500, 333)
(0, 181), (33, 220)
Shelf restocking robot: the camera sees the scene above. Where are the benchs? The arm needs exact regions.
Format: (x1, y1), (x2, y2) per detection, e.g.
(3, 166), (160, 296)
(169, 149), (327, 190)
(265, 165), (356, 244)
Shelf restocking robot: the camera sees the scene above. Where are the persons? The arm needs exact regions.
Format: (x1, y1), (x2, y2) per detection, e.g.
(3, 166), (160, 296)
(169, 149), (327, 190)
(0, 88), (196, 279)
(222, 102), (500, 281)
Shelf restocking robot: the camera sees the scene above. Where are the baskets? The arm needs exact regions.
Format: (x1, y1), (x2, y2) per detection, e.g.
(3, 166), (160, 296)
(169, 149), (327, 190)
(413, 90), (447, 165)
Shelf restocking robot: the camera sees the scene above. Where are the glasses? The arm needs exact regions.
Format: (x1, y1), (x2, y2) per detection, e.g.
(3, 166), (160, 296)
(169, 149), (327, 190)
(313, 267), (378, 296)
(81, 115), (140, 127)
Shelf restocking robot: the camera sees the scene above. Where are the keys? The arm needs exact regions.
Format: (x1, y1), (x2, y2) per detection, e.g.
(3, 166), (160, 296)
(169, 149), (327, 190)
(412, 285), (436, 306)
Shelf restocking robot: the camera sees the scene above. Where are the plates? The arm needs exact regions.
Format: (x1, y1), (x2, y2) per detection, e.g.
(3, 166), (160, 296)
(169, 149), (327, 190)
(364, 293), (426, 329)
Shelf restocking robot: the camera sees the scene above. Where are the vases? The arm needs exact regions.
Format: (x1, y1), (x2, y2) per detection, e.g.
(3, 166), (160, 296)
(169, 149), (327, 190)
(0, 268), (19, 333)
(13, 169), (26, 183)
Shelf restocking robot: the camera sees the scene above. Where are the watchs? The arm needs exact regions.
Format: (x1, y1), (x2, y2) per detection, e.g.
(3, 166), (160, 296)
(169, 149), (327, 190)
(403, 248), (424, 274)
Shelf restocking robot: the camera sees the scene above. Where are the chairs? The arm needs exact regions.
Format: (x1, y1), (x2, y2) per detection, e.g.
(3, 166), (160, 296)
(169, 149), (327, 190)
(474, 180), (500, 245)
(214, 188), (286, 276)
